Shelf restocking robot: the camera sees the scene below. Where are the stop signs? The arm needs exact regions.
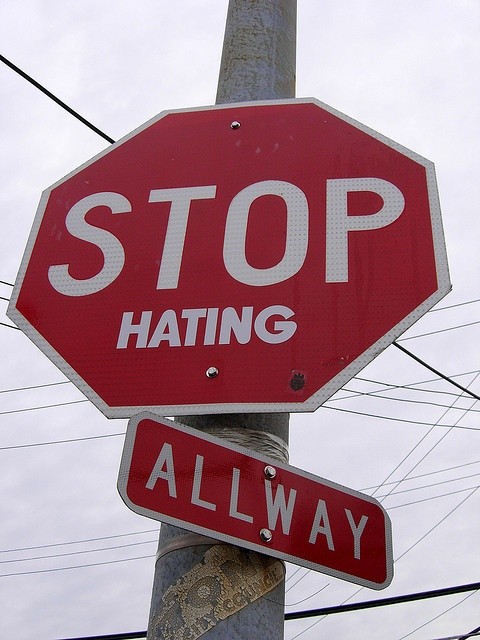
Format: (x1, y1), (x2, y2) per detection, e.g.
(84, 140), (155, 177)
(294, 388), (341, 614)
(4, 95), (453, 420)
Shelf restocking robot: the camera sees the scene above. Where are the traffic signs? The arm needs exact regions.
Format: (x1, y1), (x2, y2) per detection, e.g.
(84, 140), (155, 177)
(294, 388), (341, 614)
(116, 411), (393, 592)
